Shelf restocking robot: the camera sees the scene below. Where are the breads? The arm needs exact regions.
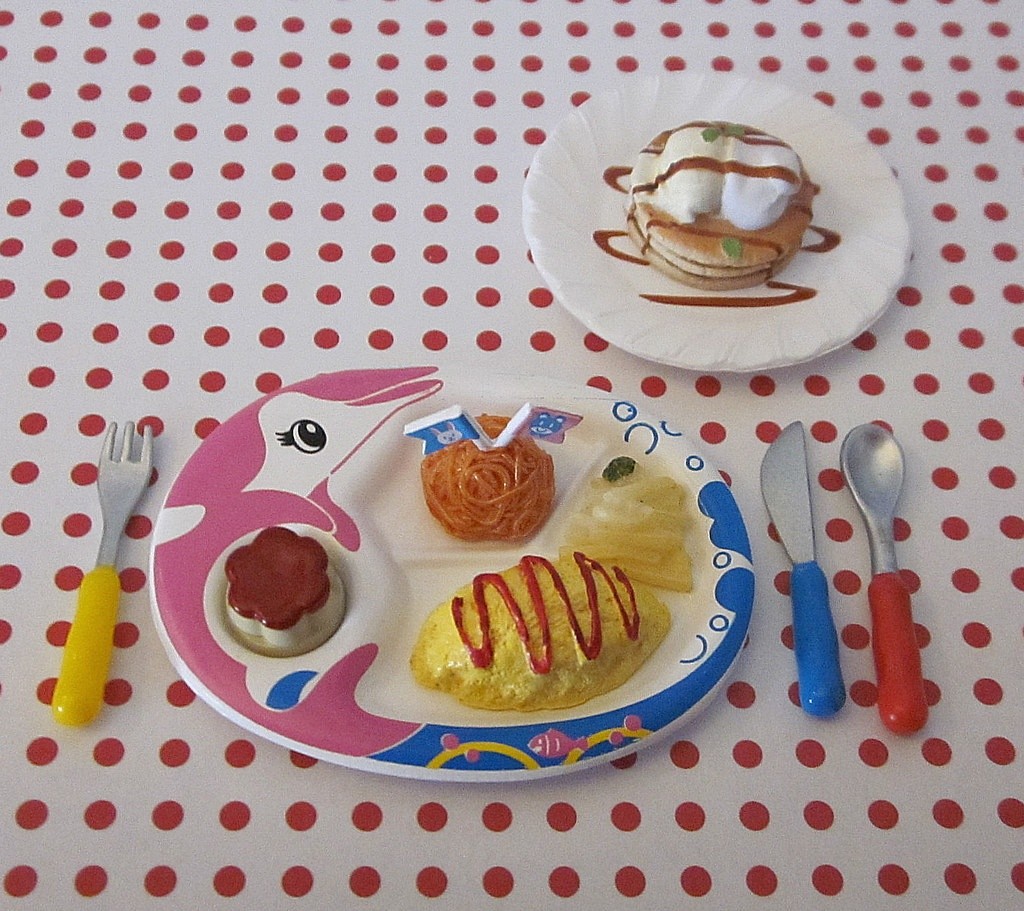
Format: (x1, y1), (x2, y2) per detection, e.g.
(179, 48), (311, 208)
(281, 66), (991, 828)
(407, 551), (671, 712)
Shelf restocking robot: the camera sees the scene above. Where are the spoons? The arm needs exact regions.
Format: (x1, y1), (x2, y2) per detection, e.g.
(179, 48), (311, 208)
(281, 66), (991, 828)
(840, 424), (930, 736)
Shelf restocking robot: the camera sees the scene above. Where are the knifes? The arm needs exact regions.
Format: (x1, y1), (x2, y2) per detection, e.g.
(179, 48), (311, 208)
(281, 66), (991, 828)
(759, 420), (847, 716)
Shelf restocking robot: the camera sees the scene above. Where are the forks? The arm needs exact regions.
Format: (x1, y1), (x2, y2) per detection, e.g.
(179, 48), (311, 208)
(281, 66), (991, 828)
(51, 422), (154, 729)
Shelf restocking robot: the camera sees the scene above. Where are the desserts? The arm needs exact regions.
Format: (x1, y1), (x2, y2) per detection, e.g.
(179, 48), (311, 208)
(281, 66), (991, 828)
(625, 120), (818, 294)
(225, 525), (345, 657)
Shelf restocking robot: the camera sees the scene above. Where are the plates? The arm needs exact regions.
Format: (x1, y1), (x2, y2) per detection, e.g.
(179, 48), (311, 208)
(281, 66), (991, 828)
(521, 71), (912, 374)
(149, 366), (756, 784)
